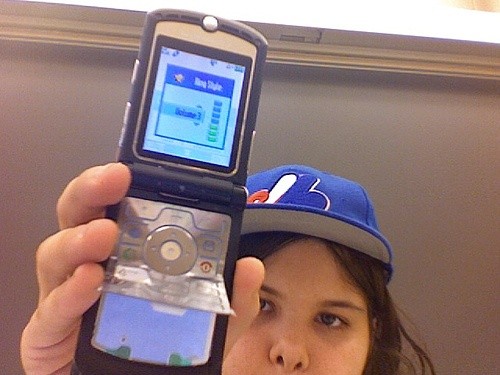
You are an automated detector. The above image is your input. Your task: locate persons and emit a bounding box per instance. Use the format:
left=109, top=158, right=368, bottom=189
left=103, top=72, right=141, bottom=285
left=20, top=161, right=435, bottom=375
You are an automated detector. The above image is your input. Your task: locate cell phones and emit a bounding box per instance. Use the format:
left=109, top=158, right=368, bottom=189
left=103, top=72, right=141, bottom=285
left=73, top=8, right=268, bottom=374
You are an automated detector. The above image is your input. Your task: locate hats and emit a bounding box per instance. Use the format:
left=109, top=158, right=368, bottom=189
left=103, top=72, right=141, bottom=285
left=240, top=165, right=392, bottom=265
